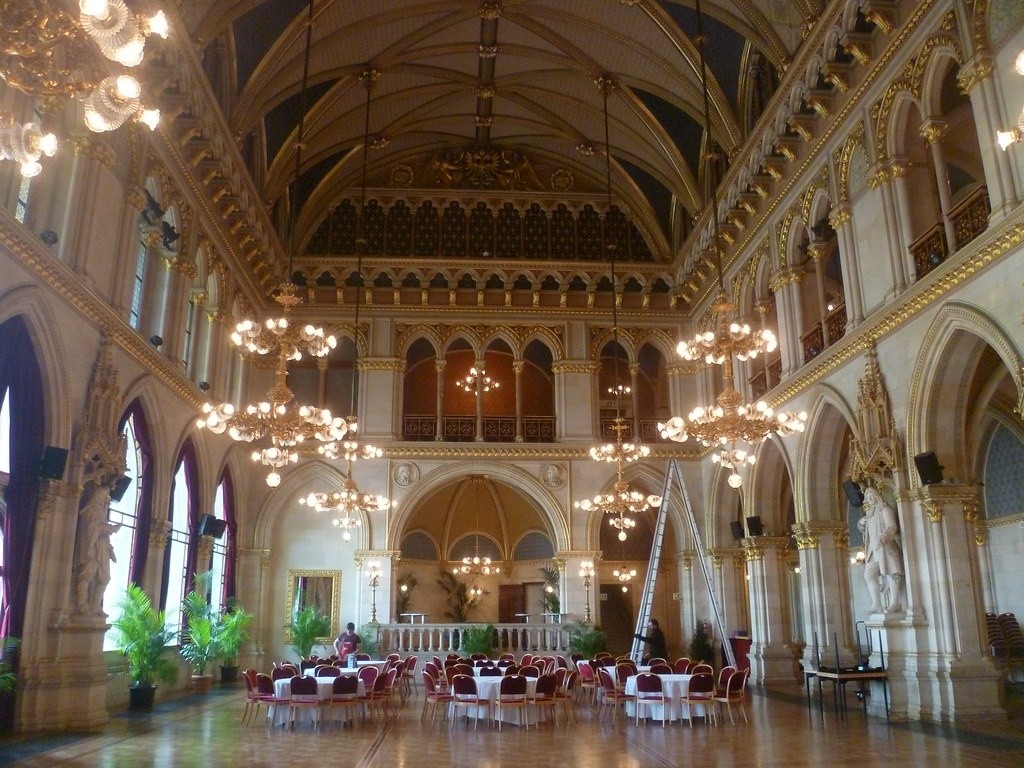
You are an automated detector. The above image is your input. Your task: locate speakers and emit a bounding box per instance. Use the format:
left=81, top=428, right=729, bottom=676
left=38, top=446, right=69, bottom=481
left=746, top=516, right=763, bottom=536
left=197, top=514, right=227, bottom=540
left=109, top=475, right=132, bottom=503
left=842, top=479, right=865, bottom=508
left=914, top=451, right=944, bottom=486
left=730, top=521, right=744, bottom=541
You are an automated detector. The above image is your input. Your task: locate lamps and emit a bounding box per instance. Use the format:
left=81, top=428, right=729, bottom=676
left=653, top=320, right=808, bottom=495
left=452, top=557, right=507, bottom=596
left=301, top=413, right=396, bottom=542
left=198, top=317, right=348, bottom=489
left=453, top=363, right=497, bottom=401
left=0, top=1, right=170, bottom=179
left=996, top=46, right=1024, bottom=157
left=573, top=440, right=663, bottom=544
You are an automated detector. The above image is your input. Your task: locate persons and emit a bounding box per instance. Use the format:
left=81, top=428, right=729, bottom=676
left=633, top=619, right=669, bottom=666
left=333, top=622, right=362, bottom=662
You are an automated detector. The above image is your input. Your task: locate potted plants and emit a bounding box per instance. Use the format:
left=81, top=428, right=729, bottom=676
left=112, top=583, right=726, bottom=715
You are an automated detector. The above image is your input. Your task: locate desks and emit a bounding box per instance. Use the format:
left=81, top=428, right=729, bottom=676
left=472, top=659, right=503, bottom=666
left=344, top=661, right=387, bottom=676
left=797, top=630, right=893, bottom=728
left=576, top=659, right=593, bottom=669
left=469, top=667, right=511, bottom=676
left=625, top=676, right=726, bottom=718
left=301, top=667, right=363, bottom=678
left=597, top=665, right=650, bottom=705
left=449, top=676, right=557, bottom=724
left=271, top=677, right=365, bottom=726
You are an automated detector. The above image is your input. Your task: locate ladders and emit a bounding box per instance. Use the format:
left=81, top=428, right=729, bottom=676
left=629, top=457, right=737, bottom=668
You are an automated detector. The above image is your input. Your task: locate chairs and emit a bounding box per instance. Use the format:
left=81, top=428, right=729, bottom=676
left=244, top=653, right=750, bottom=732
